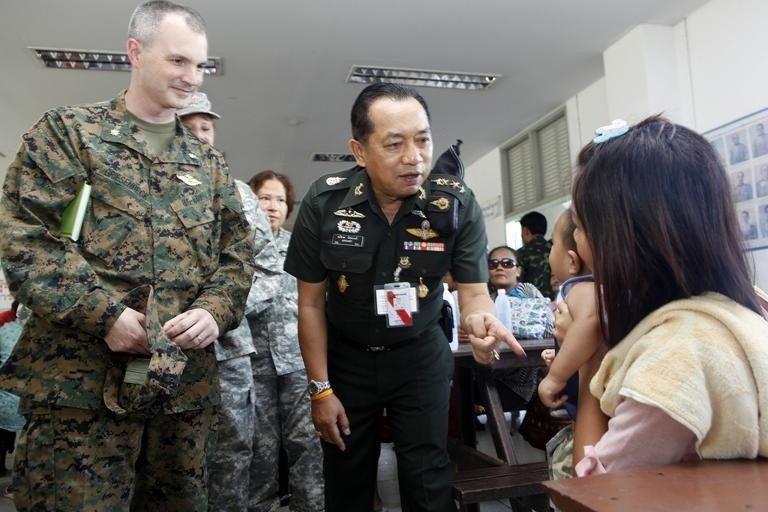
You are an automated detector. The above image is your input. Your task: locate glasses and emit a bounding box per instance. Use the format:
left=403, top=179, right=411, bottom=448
left=488, top=258, right=521, bottom=270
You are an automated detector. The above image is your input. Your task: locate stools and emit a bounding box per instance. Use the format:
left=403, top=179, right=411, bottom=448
left=445, top=459, right=561, bottom=511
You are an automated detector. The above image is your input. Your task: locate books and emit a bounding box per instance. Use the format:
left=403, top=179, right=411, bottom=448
left=60, top=183, right=92, bottom=242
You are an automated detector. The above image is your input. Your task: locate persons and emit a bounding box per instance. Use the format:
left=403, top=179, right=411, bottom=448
left=283, top=80, right=527, bottom=512
left=175, top=90, right=283, bottom=512
left=245, top=168, right=327, bottom=512
left=1, top=298, right=33, bottom=500
left=440, top=109, right=768, bottom=511
left=0, top=0, right=255, bottom=511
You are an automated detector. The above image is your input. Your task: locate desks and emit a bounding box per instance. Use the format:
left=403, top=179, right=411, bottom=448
left=447, top=335, right=561, bottom=465
left=542, top=460, right=768, bottom=512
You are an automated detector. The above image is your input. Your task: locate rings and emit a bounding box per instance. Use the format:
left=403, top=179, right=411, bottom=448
left=197, top=334, right=204, bottom=343
left=314, top=430, right=322, bottom=437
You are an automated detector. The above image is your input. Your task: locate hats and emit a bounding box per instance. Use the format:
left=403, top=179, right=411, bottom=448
left=176, top=91, right=221, bottom=121
left=103, top=284, right=186, bottom=418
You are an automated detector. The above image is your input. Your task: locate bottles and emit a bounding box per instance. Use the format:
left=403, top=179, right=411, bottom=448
left=493, top=288, right=513, bottom=335
left=441, top=282, right=458, bottom=353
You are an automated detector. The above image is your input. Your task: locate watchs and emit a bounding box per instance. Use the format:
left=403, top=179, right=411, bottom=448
left=307, top=378, right=332, bottom=396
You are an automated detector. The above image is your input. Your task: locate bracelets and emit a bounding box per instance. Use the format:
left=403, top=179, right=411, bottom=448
left=309, top=388, right=333, bottom=401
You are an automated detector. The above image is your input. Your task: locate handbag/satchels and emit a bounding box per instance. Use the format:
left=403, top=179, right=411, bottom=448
left=504, top=296, right=551, bottom=339
